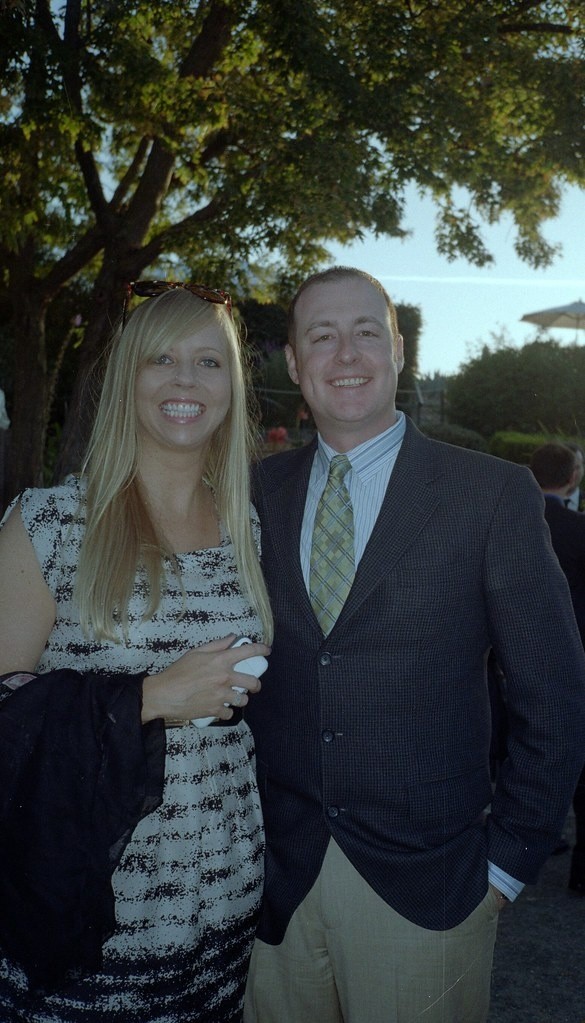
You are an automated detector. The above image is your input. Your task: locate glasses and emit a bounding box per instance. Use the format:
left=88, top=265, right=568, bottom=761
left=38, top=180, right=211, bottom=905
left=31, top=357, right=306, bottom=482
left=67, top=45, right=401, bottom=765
left=121, top=279, right=233, bottom=332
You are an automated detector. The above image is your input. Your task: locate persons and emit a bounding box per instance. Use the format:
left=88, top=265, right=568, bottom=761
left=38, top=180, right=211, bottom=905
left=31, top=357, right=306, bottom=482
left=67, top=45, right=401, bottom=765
left=244, top=266, right=585, bottom=1023
left=521, top=444, right=585, bottom=650
left=0, top=289, right=268, bottom=1023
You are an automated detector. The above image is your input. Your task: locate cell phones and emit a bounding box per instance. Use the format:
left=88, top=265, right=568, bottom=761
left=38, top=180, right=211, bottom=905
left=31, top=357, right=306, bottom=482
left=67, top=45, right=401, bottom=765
left=190, top=635, right=269, bottom=729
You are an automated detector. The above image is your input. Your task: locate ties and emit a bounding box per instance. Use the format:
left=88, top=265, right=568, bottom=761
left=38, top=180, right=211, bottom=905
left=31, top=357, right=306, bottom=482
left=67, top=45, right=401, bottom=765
left=563, top=500, right=571, bottom=508
left=308, top=454, right=353, bottom=640
